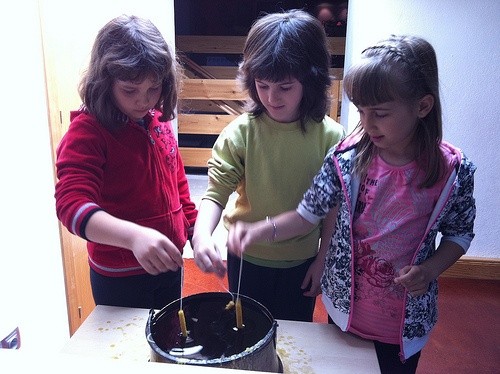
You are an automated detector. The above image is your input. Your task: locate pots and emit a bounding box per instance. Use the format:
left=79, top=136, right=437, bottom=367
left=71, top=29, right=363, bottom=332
left=145, top=291, right=280, bottom=373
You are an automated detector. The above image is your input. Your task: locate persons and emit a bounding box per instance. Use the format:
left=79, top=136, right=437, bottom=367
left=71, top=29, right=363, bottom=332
left=228, top=34, right=477, bottom=374
left=191, top=5, right=348, bottom=322
left=53, top=13, right=206, bottom=308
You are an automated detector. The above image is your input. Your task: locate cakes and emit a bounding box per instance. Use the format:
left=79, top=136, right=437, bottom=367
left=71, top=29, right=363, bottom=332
left=146, top=292, right=279, bottom=374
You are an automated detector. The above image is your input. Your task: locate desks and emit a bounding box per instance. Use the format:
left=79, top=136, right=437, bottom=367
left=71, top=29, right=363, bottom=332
left=67, top=304, right=384, bottom=374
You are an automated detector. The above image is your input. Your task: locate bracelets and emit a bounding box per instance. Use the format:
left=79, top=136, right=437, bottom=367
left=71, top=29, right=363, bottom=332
left=267, top=216, right=278, bottom=241
